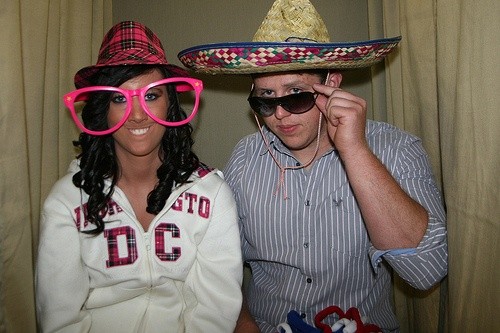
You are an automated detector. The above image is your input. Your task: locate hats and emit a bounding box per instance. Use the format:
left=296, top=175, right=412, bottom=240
left=177, top=0, right=403, bottom=74
left=73, top=20, right=192, bottom=96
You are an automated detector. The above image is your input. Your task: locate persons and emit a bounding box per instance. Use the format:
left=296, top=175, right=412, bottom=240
left=34, top=20, right=243, bottom=333
left=178, top=0, right=448, bottom=333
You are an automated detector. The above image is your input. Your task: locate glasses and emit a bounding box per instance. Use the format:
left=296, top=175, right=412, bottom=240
left=247, top=74, right=326, bottom=116
left=64, top=77, right=203, bottom=133
